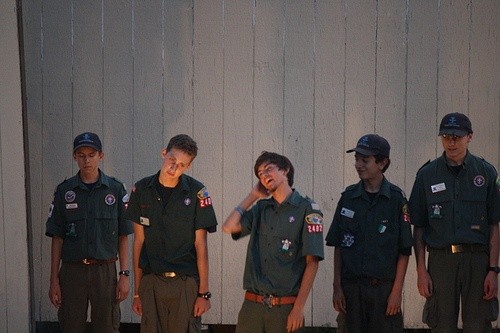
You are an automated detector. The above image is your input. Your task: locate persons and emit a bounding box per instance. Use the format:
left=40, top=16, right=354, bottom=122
left=222, top=151, right=324, bottom=333
left=125, top=134, right=217, bottom=333
left=325, top=134, right=413, bottom=333
left=410, top=112, right=500, bottom=333
left=44, top=132, right=134, bottom=333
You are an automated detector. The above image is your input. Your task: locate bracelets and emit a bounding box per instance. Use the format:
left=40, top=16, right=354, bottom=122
left=236, top=207, right=244, bottom=217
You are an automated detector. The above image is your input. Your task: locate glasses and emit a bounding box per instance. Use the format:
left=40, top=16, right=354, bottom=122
left=440, top=134, right=465, bottom=141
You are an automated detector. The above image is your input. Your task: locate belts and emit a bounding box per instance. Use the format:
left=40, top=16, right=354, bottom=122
left=80, top=257, right=118, bottom=265
left=245, top=292, right=296, bottom=305
left=142, top=269, right=198, bottom=277
left=441, top=243, right=483, bottom=253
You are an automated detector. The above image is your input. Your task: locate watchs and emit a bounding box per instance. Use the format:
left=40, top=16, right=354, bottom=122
left=119, top=270, right=129, bottom=276
left=489, top=266, right=499, bottom=273
left=197, top=292, right=211, bottom=299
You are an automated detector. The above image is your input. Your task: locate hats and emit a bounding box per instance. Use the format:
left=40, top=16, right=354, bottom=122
left=438, top=112, right=472, bottom=136
left=72, top=132, right=103, bottom=151
left=346, top=134, right=390, bottom=156
